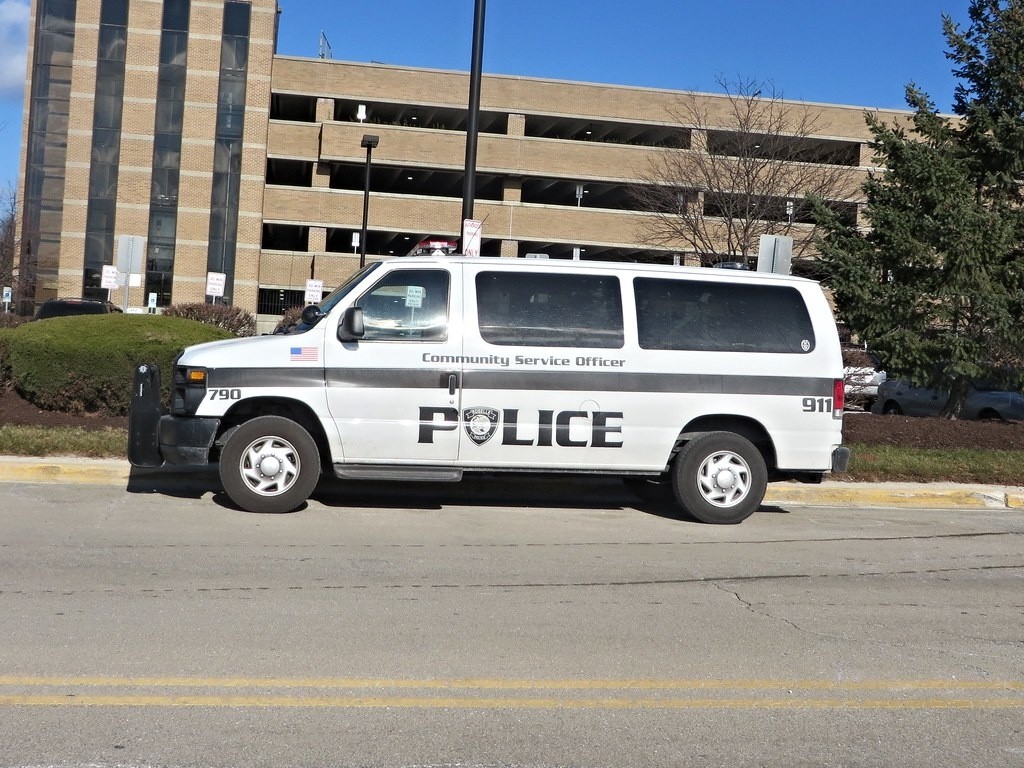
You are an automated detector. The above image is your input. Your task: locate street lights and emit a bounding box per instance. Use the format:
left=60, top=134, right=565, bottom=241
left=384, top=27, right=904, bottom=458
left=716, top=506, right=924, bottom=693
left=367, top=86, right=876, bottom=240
left=359, top=134, right=380, bottom=268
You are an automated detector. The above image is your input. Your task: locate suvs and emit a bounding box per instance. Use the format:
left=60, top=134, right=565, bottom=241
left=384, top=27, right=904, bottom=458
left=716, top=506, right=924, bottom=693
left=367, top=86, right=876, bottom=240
left=31, top=296, right=124, bottom=320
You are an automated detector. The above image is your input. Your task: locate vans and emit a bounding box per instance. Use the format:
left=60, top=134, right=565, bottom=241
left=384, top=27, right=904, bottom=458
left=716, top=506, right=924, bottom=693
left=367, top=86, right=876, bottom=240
left=126, top=255, right=852, bottom=525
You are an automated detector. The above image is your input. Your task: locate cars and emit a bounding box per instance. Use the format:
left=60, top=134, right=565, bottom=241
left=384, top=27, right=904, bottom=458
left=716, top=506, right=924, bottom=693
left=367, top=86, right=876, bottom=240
left=841, top=346, right=1024, bottom=422
